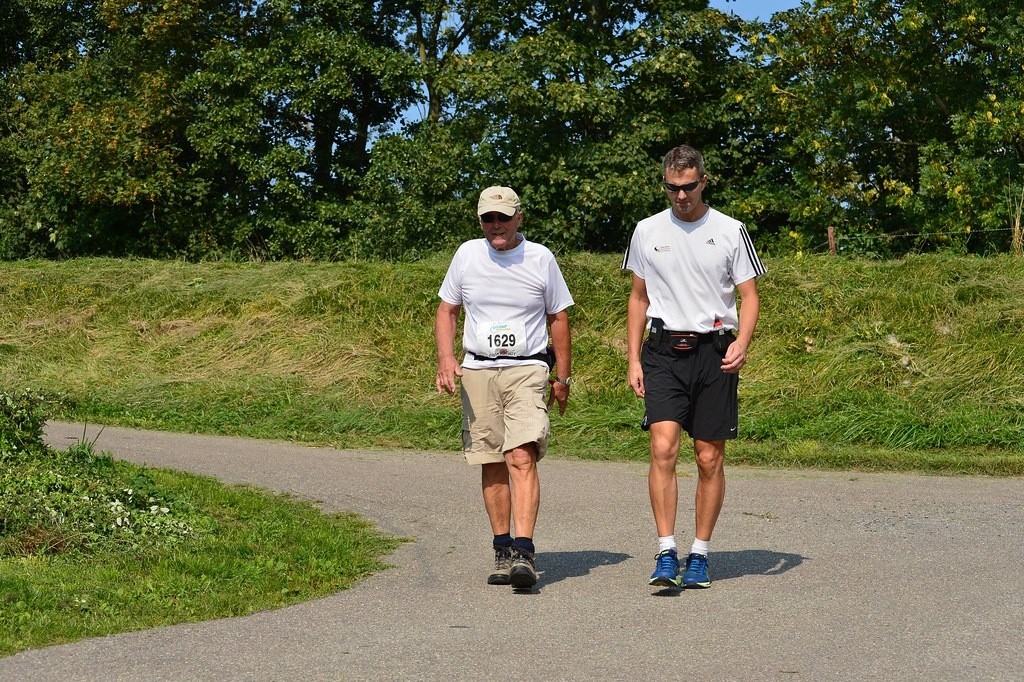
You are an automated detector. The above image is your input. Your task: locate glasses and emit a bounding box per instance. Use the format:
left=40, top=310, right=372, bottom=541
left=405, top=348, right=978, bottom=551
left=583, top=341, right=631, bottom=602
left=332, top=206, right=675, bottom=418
left=662, top=176, right=702, bottom=191
left=479, top=213, right=513, bottom=223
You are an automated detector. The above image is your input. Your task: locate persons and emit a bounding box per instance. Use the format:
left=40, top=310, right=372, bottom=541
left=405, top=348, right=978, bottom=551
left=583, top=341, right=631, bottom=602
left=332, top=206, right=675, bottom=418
left=620, top=146, right=767, bottom=589
left=434, top=187, right=575, bottom=589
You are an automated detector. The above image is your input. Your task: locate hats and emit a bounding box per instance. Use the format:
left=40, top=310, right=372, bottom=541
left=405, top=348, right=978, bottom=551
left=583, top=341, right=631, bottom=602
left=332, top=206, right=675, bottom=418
left=476, top=186, right=521, bottom=217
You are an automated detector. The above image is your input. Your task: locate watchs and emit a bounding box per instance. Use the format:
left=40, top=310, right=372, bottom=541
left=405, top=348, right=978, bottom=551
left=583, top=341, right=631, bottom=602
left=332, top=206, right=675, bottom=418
left=555, top=376, right=572, bottom=385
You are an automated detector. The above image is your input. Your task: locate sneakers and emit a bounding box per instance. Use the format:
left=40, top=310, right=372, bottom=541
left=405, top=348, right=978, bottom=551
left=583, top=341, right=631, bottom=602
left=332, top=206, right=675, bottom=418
left=488, top=542, right=514, bottom=585
left=647, top=547, right=682, bottom=587
left=509, top=544, right=537, bottom=588
left=681, top=552, right=709, bottom=588
